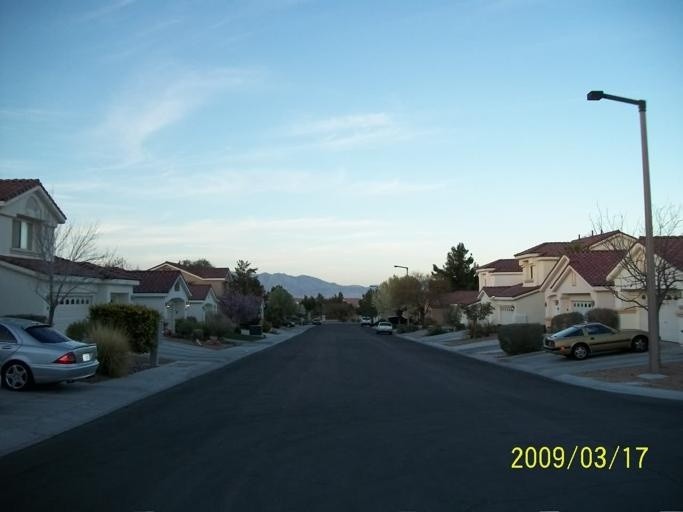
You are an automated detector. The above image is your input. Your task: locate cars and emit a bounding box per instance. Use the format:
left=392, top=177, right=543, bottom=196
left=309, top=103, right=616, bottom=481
left=376, top=322, right=393, bottom=335
left=0, top=317, right=101, bottom=391
left=541, top=322, right=649, bottom=360
left=287, top=315, right=303, bottom=324
left=311, top=317, right=321, bottom=325
left=280, top=318, right=294, bottom=328
left=360, top=317, right=373, bottom=327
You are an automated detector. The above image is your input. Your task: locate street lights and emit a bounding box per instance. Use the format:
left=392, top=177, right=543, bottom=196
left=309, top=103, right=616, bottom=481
left=394, top=265, right=410, bottom=330
left=370, top=285, right=379, bottom=294
left=584, top=89, right=664, bottom=373
